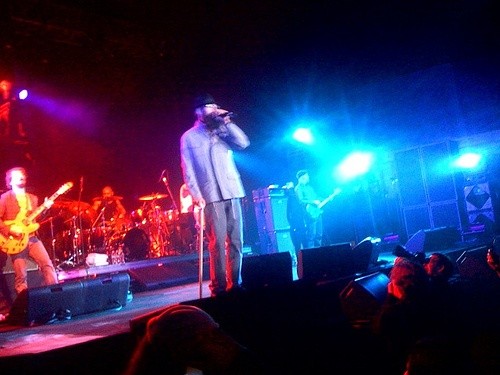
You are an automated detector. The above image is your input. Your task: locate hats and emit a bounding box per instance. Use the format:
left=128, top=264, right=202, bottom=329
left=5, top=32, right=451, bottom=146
left=192, top=95, right=215, bottom=111
left=296, top=169, right=308, bottom=179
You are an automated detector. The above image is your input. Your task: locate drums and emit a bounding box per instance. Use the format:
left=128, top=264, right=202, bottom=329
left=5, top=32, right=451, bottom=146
left=108, top=227, right=151, bottom=262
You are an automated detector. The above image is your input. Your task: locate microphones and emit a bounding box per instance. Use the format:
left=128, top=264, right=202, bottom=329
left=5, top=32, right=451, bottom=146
left=158, top=170, right=164, bottom=183
left=210, top=112, right=234, bottom=120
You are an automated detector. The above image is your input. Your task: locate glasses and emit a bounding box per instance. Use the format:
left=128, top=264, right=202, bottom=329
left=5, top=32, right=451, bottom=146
left=204, top=103, right=221, bottom=109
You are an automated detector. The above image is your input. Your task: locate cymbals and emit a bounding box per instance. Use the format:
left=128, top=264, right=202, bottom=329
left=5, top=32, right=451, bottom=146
left=139, top=195, right=168, bottom=200
left=93, top=196, right=123, bottom=201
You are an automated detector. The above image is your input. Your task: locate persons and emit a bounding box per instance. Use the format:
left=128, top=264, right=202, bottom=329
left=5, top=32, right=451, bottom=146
left=93, top=186, right=126, bottom=220
left=0, top=167, right=60, bottom=294
left=128, top=236, right=500, bottom=375
left=181, top=93, right=251, bottom=297
left=294, top=169, right=324, bottom=248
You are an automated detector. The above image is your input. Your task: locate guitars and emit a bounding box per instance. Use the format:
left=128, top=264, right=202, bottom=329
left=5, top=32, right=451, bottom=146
left=0, top=180, right=74, bottom=255
left=306, top=187, right=343, bottom=220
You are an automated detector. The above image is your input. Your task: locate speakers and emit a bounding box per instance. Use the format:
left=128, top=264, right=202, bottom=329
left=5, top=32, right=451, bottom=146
left=351, top=236, right=383, bottom=269
left=404, top=225, right=457, bottom=253
left=26, top=272, right=130, bottom=322
left=238, top=252, right=293, bottom=287
left=295, top=243, right=358, bottom=282
left=254, top=198, right=299, bottom=281
